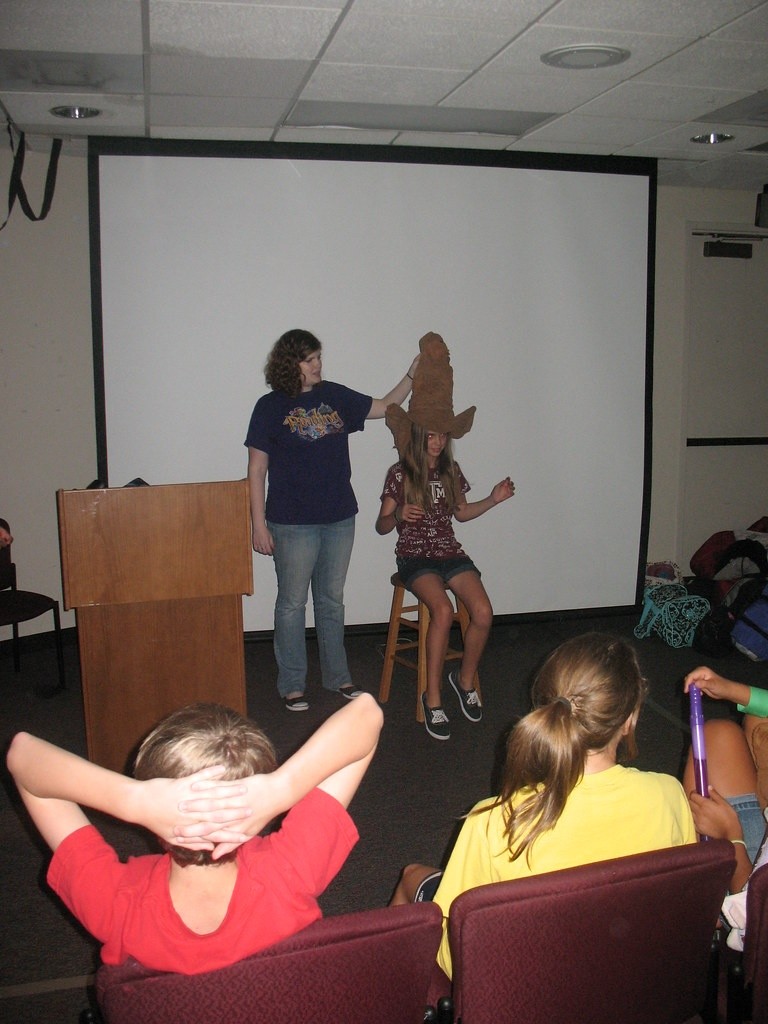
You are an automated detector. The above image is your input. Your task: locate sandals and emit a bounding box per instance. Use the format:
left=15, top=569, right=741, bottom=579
left=284, top=694, right=309, bottom=711
left=332, top=686, right=364, bottom=700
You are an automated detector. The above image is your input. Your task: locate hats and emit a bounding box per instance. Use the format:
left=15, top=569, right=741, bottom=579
left=385, top=331, right=477, bottom=461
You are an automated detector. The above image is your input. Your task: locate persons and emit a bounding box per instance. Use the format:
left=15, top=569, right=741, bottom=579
left=374, top=395, right=516, bottom=741
left=389, top=631, right=696, bottom=1007
left=684, top=664, right=768, bottom=952
left=8, top=689, right=385, bottom=975
left=244, top=328, right=422, bottom=711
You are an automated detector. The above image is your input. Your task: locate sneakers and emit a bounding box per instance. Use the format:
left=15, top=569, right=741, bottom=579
left=422, top=691, right=451, bottom=740
left=448, top=670, right=482, bottom=723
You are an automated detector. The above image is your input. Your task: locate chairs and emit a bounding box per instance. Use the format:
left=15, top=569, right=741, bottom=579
left=0, top=517, right=65, bottom=688
left=80, top=838, right=768, bottom=1024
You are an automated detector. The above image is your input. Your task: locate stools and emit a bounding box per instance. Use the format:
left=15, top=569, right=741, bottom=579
left=379, top=572, right=483, bottom=723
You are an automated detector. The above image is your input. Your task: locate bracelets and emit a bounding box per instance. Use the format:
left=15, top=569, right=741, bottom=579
left=729, top=838, right=748, bottom=850
left=392, top=506, right=400, bottom=523
left=406, top=373, right=414, bottom=381
